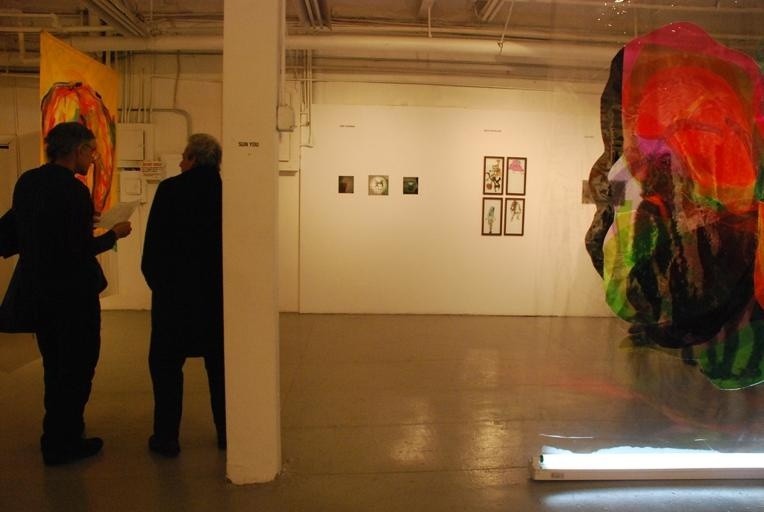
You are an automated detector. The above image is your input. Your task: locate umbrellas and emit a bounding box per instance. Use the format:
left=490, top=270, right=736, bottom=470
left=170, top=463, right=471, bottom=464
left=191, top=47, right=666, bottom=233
left=43, top=437, right=103, bottom=467
left=149, top=435, right=181, bottom=458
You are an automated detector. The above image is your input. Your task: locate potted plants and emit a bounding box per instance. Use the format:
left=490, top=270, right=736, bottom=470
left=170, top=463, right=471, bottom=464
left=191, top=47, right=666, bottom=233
left=506, top=157, right=526, bottom=196
left=483, top=156, right=503, bottom=195
left=482, top=197, right=502, bottom=235
left=504, top=198, right=525, bottom=236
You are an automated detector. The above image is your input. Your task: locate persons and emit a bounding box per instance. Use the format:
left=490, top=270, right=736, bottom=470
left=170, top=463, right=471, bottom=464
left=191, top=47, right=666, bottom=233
left=138, top=131, right=229, bottom=456
left=9, top=121, right=133, bottom=465
